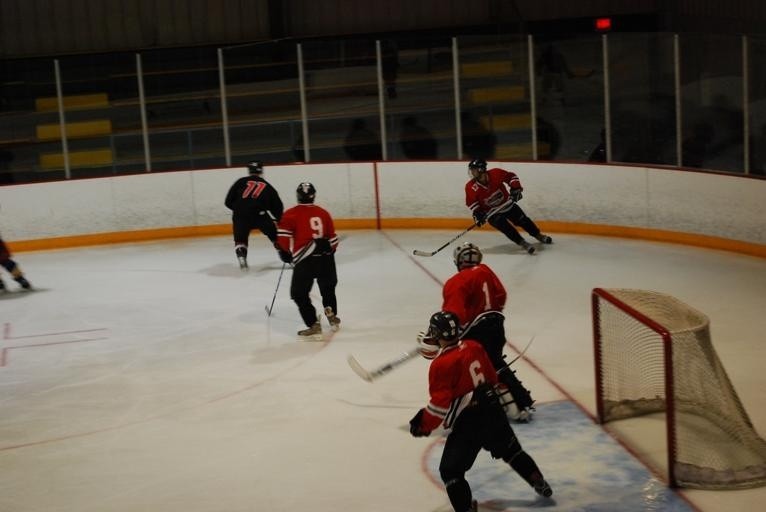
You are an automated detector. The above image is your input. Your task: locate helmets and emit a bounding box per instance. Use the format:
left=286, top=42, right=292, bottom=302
left=469, top=159, right=487, bottom=173
left=428, top=311, right=458, bottom=342
left=453, top=243, right=482, bottom=272
left=296, top=183, right=316, bottom=201
left=249, top=160, right=263, bottom=174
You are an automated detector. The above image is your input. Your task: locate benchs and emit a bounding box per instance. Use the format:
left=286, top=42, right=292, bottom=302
left=2, top=33, right=552, bottom=183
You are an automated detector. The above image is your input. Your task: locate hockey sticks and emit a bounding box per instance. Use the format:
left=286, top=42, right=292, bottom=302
left=414, top=198, right=513, bottom=258
left=494, top=339, right=535, bottom=367
left=343, top=348, right=420, bottom=384
left=264, top=261, right=287, bottom=317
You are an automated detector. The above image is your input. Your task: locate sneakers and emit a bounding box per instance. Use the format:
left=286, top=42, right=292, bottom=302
left=535, top=481, right=553, bottom=497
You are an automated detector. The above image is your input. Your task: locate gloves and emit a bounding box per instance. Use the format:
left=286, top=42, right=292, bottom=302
left=416, top=332, right=441, bottom=360
left=279, top=249, right=293, bottom=264
left=508, top=189, right=522, bottom=202
left=410, top=411, right=430, bottom=437
left=473, top=211, right=488, bottom=227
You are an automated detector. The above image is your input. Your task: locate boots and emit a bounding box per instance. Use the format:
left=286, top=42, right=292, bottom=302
left=298, top=315, right=322, bottom=336
left=15, top=275, right=30, bottom=289
left=324, top=307, right=341, bottom=325
left=536, top=234, right=551, bottom=244
left=518, top=240, right=535, bottom=254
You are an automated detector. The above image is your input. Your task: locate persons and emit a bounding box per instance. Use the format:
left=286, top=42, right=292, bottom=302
left=430, top=241, right=535, bottom=425
left=276, top=182, right=342, bottom=338
left=402, top=313, right=556, bottom=511
left=1, top=239, right=32, bottom=298
left=223, top=158, right=293, bottom=269
left=463, top=158, right=552, bottom=257
